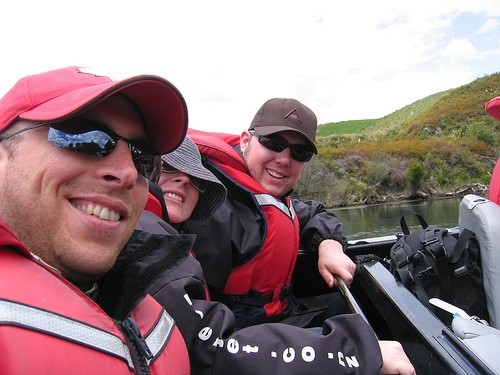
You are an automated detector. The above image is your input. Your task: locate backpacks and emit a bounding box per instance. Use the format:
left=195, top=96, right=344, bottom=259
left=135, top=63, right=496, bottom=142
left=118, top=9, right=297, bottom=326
left=389, top=211, right=490, bottom=328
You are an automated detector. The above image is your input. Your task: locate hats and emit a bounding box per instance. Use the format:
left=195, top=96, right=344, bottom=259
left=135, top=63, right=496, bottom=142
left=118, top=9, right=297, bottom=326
left=159, top=135, right=228, bottom=222
left=248, top=97, right=318, bottom=156
left=0, top=65, right=188, bottom=154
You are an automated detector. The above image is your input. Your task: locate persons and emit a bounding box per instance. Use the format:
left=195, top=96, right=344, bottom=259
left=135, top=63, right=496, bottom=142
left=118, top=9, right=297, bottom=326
left=145, top=134, right=418, bottom=375
left=186, top=98, right=356, bottom=328
left=0, top=64, right=214, bottom=375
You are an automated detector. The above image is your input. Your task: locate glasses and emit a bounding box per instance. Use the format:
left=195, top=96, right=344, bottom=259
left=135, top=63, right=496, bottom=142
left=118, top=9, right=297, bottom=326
left=160, top=161, right=208, bottom=194
left=0, top=119, right=162, bottom=185
left=249, top=129, right=314, bottom=163
